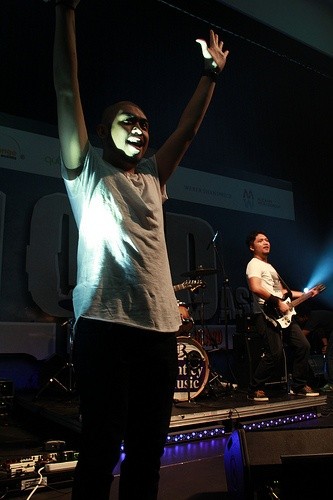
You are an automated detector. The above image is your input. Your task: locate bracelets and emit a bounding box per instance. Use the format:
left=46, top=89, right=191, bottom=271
left=203, top=68, right=218, bottom=82
left=323, top=345, right=326, bottom=346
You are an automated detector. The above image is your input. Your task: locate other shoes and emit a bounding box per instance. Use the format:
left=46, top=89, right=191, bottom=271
left=290, top=385, right=319, bottom=397
left=246, top=390, right=269, bottom=401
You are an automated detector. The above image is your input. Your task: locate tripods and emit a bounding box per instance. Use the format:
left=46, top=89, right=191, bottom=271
left=36, top=317, right=75, bottom=399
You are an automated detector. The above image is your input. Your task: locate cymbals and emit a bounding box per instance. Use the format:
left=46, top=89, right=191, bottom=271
left=59, top=298, right=74, bottom=312
left=185, top=301, right=209, bottom=305
left=182, top=267, right=218, bottom=277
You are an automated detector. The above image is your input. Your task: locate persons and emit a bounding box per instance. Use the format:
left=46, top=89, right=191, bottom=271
left=246, top=233, right=320, bottom=400
left=296, top=311, right=328, bottom=395
left=50, top=0, right=229, bottom=500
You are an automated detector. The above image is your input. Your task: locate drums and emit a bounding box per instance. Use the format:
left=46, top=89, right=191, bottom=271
left=171, top=336, right=209, bottom=402
left=186, top=327, right=212, bottom=347
left=175, top=301, right=195, bottom=335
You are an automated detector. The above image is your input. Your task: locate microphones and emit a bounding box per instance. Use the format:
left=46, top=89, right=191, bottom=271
left=213, top=231, right=219, bottom=241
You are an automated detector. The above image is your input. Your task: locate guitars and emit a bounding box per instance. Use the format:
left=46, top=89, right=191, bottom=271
left=262, top=282, right=327, bottom=329
left=172, top=279, right=208, bottom=292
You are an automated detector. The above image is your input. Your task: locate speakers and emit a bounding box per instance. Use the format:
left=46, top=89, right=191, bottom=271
left=232, top=333, right=289, bottom=387
